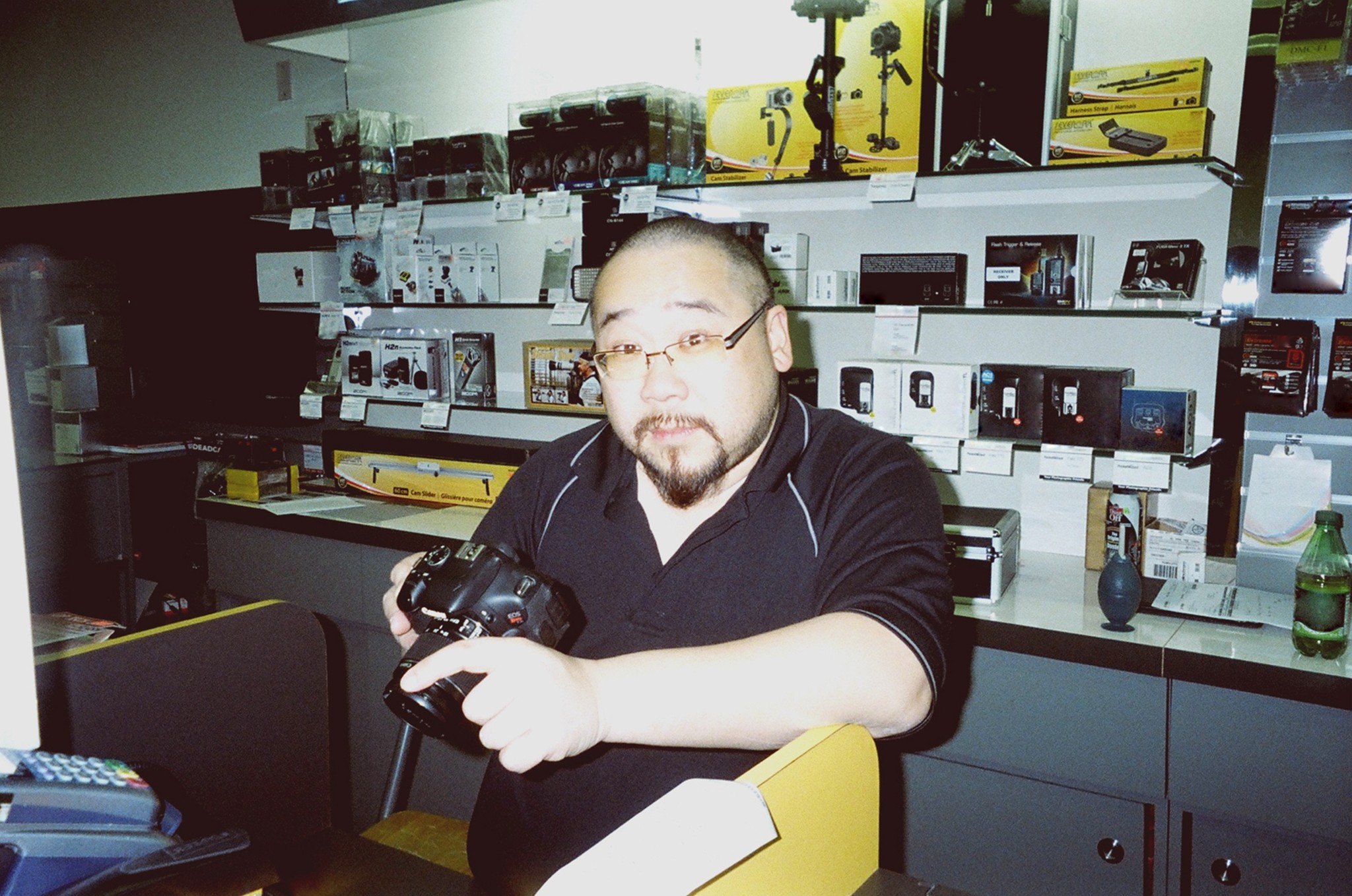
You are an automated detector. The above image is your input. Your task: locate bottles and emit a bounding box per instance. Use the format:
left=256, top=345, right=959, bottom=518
left=1291, top=510, right=1352, bottom=660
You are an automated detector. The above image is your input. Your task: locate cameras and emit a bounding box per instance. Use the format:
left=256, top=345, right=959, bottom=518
left=766, top=88, right=793, bottom=108
left=851, top=89, right=863, bottom=99
left=382, top=539, right=572, bottom=751
left=871, top=22, right=900, bottom=49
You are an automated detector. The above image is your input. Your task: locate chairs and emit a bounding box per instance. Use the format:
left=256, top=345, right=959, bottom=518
left=355, top=719, right=495, bottom=896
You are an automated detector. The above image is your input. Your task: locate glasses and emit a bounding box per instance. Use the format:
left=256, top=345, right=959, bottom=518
left=592, top=297, right=775, bottom=381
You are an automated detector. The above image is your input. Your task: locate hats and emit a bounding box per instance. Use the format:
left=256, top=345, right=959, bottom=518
left=580, top=351, right=594, bottom=361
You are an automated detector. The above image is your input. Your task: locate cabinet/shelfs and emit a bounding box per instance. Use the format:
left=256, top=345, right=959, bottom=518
left=251, top=155, right=1246, bottom=467
left=203, top=522, right=1352, bottom=896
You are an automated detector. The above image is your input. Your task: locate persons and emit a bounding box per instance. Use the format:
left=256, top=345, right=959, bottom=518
left=382, top=218, right=956, bottom=894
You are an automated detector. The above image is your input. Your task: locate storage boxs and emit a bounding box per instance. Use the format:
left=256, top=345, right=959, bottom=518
left=1049, top=106, right=1213, bottom=167
left=1066, top=57, right=1213, bottom=118
